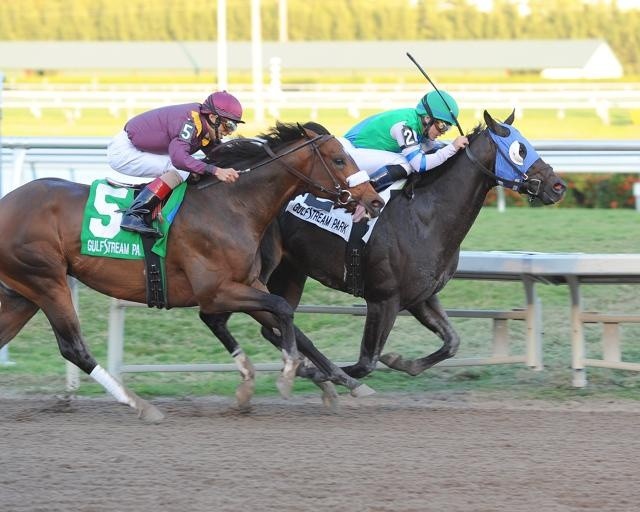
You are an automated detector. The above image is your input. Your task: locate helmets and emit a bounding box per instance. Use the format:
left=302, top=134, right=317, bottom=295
left=416, top=90, right=459, bottom=127
left=200, top=91, right=246, bottom=123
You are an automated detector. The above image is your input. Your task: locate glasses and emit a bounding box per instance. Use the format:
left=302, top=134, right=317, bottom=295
left=217, top=119, right=241, bottom=131
left=435, top=121, right=449, bottom=134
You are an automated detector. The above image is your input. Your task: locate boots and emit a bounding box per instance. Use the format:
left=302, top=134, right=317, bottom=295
left=120, top=178, right=172, bottom=237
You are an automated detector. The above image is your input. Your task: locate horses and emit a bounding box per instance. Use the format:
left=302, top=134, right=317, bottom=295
left=0, top=119, right=385, bottom=425
left=197, top=106, right=566, bottom=407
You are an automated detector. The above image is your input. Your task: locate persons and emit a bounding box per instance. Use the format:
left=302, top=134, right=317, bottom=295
left=336, top=90, right=469, bottom=194
left=106, top=92, right=244, bottom=238
